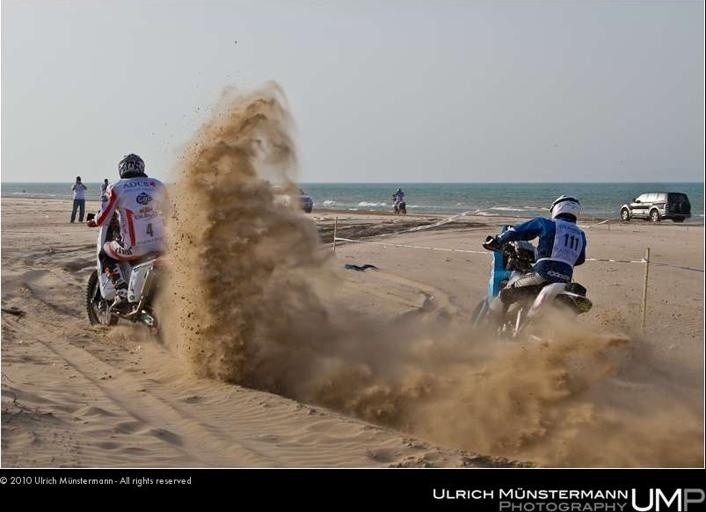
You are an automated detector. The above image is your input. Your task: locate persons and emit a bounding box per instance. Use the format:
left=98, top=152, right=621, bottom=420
left=70, top=176, right=88, bottom=223
left=99, top=178, right=110, bottom=208
left=391, top=187, right=406, bottom=213
left=86, top=152, right=175, bottom=312
left=481, top=194, right=589, bottom=332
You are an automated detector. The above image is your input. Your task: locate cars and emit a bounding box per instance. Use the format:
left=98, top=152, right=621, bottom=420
left=272, top=186, right=313, bottom=213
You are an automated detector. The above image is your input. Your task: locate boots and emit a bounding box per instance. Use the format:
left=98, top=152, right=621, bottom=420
left=105, top=264, right=128, bottom=313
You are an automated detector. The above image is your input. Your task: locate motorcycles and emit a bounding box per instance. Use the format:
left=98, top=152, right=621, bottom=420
left=466, top=224, right=592, bottom=347
left=391, top=198, right=406, bottom=215
left=86, top=213, right=173, bottom=347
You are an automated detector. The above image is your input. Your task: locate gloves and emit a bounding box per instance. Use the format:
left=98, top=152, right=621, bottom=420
left=87, top=219, right=98, bottom=227
left=483, top=236, right=500, bottom=252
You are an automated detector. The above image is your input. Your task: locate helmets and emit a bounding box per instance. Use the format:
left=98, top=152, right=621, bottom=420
left=119, top=154, right=145, bottom=178
left=550, top=194, right=583, bottom=220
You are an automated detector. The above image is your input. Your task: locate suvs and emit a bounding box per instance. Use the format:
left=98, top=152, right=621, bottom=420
left=620, top=192, right=692, bottom=222
